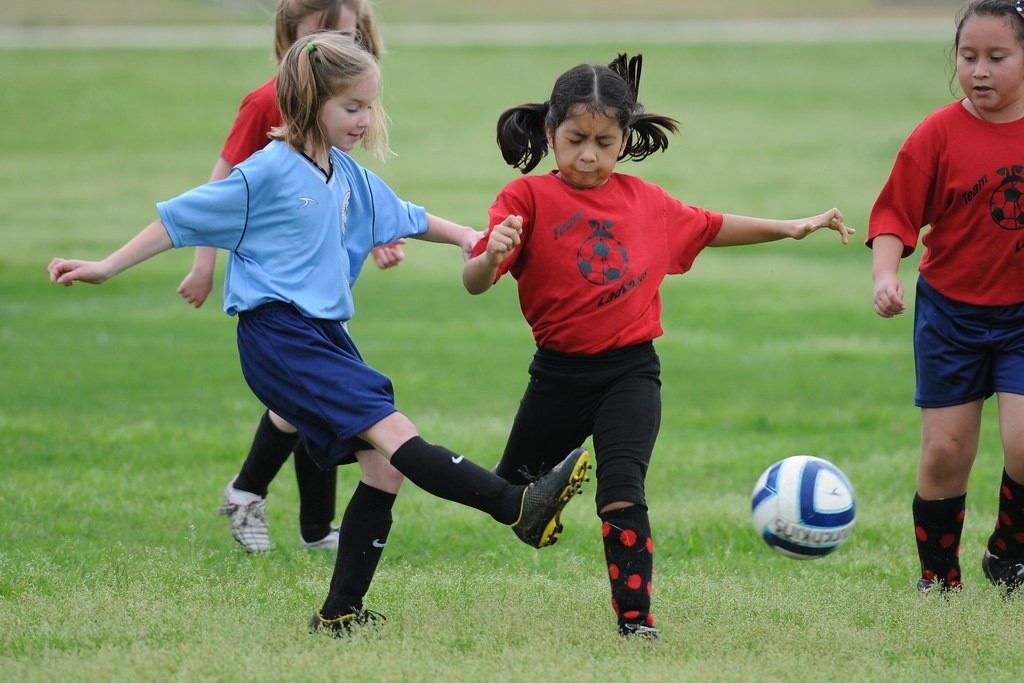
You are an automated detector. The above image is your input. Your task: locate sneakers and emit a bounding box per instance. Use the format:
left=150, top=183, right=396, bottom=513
left=510, top=449, right=591, bottom=549
left=618, top=624, right=659, bottom=642
left=917, top=581, right=962, bottom=610
left=300, top=526, right=339, bottom=550
left=983, top=547, right=1024, bottom=601
left=307, top=609, right=386, bottom=640
left=216, top=476, right=271, bottom=552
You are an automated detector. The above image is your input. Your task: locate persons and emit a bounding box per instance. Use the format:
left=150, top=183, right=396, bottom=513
left=49, top=32, right=593, bottom=638
left=864, top=0, right=1024, bottom=599
left=178, top=0, right=403, bottom=554
left=463, top=54, right=855, bottom=640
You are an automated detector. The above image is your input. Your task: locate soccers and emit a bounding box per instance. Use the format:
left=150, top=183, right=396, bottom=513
left=750, top=454, right=855, bottom=562
